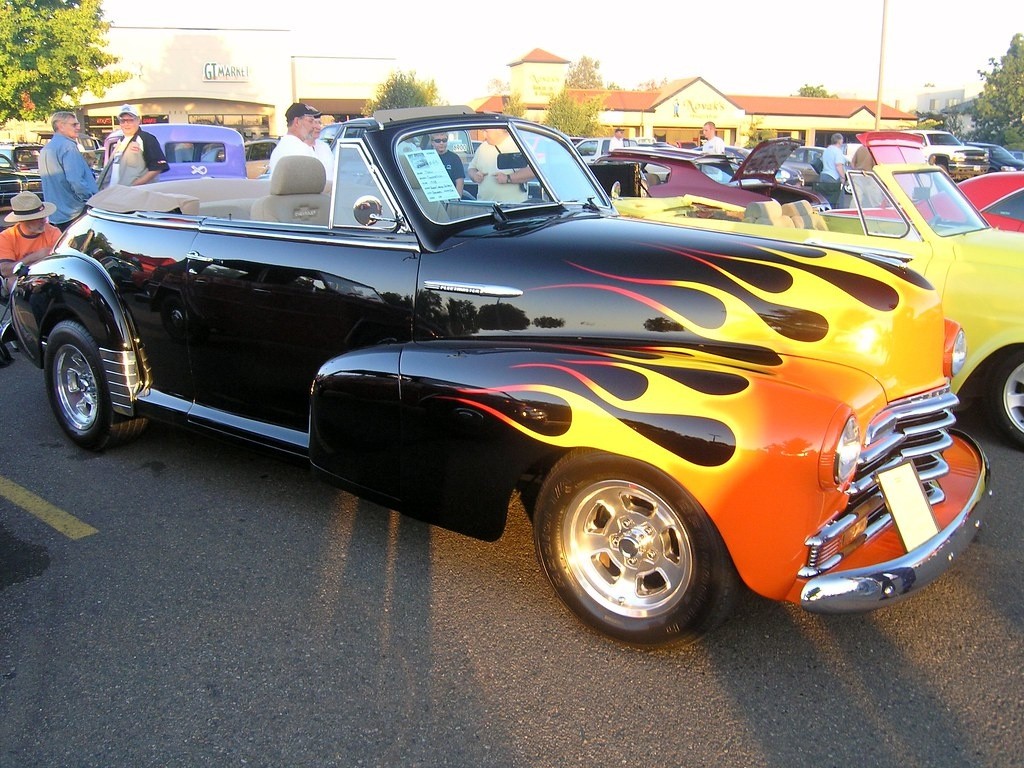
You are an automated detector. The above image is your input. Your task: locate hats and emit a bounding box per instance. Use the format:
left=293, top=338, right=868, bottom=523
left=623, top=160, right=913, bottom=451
left=4, top=191, right=57, bottom=222
left=615, top=128, right=624, bottom=133
left=118, top=104, right=142, bottom=118
left=285, top=102, right=322, bottom=122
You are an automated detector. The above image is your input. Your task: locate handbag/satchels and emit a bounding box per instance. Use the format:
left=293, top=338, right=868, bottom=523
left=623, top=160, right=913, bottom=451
left=809, top=152, right=823, bottom=174
left=520, top=182, right=528, bottom=193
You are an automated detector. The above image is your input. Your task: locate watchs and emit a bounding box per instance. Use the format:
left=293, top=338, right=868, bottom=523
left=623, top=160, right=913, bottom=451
left=843, top=178, right=846, bottom=179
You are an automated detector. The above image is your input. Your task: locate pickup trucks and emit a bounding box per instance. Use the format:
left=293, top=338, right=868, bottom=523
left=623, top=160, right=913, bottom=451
left=575, top=138, right=654, bottom=164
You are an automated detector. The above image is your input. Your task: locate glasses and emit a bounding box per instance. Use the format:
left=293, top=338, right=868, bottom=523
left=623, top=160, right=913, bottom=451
left=64, top=122, right=79, bottom=128
left=433, top=139, right=447, bottom=143
left=119, top=119, right=135, bottom=124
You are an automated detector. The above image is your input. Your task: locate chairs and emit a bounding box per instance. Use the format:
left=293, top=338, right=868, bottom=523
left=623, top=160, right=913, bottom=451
left=402, top=156, right=450, bottom=227
left=744, top=200, right=796, bottom=229
left=784, top=200, right=828, bottom=231
left=253, top=154, right=332, bottom=227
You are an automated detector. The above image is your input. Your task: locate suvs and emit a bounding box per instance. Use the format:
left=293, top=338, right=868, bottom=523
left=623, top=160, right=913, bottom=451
left=897, top=130, right=990, bottom=181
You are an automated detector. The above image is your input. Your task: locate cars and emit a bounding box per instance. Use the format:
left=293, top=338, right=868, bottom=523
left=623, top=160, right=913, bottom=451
left=965, top=143, right=1024, bottom=172
left=586, top=136, right=832, bottom=213
left=781, top=146, right=827, bottom=186
left=0, top=143, right=45, bottom=212
left=103, top=123, right=247, bottom=188
left=692, top=146, right=751, bottom=165
left=1008, top=151, right=1024, bottom=161
left=200, top=139, right=281, bottom=179
left=827, top=130, right=1024, bottom=232
left=608, top=160, right=1024, bottom=450
left=8, top=114, right=993, bottom=646
left=29, top=129, right=104, bottom=151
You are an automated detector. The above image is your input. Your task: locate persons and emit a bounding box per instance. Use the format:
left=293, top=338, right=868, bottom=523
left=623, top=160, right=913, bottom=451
left=609, top=128, right=625, bottom=152
left=269, top=103, right=337, bottom=180
left=468, top=129, right=536, bottom=203
left=809, top=133, right=877, bottom=210
left=37, top=112, right=98, bottom=231
left=108, top=104, right=170, bottom=187
left=702, top=122, right=725, bottom=182
left=0, top=191, right=62, bottom=300
left=429, top=131, right=465, bottom=201
